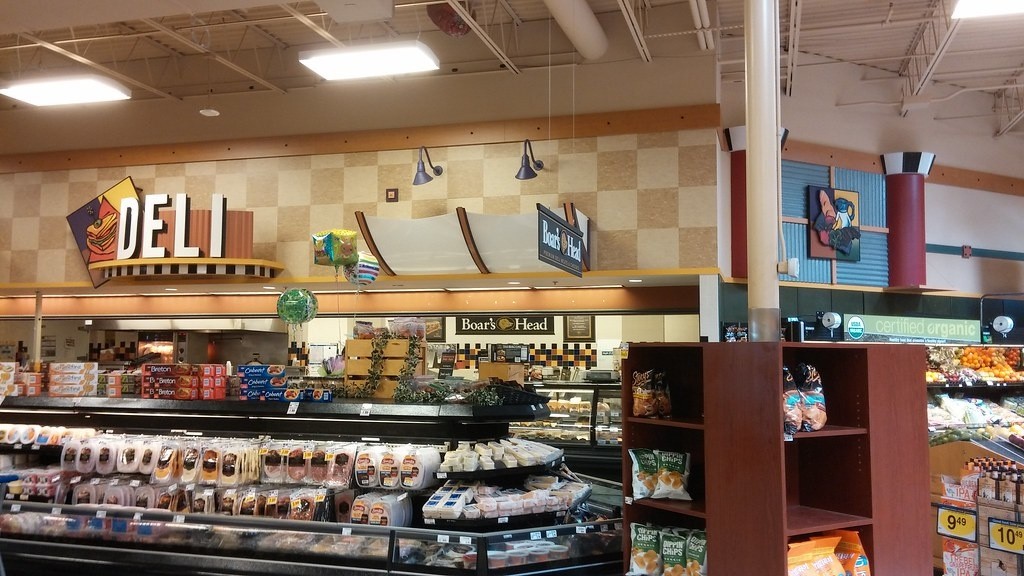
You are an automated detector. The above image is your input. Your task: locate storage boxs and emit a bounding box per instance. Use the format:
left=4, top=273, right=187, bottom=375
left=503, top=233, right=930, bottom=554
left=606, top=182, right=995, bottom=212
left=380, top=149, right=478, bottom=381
left=0, top=361, right=332, bottom=395
left=488, top=344, right=530, bottom=363
left=939, top=467, right=1024, bottom=576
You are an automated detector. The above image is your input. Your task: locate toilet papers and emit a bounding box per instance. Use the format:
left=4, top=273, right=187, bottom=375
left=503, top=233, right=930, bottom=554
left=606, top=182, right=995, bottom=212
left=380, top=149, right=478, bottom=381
left=993, top=316, right=1014, bottom=334
left=823, top=311, right=841, bottom=330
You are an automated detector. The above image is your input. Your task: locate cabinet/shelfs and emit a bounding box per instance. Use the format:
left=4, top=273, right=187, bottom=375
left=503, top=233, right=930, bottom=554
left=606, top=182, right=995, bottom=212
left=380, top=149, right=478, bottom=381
left=345, top=338, right=427, bottom=400
left=0, top=395, right=621, bottom=576
left=620, top=341, right=933, bottom=576
left=507, top=380, right=623, bottom=484
left=926, top=380, right=1024, bottom=437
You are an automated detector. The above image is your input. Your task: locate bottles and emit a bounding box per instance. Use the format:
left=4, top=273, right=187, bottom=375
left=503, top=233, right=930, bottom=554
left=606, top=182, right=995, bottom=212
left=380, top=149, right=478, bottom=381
left=966, top=455, right=1024, bottom=505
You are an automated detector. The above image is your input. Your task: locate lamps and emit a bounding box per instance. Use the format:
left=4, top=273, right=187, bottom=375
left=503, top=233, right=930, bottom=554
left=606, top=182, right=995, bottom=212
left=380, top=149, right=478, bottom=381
left=412, top=146, right=443, bottom=186
left=515, top=139, right=543, bottom=180
left=298, top=7, right=440, bottom=81
left=0, top=22, right=132, bottom=108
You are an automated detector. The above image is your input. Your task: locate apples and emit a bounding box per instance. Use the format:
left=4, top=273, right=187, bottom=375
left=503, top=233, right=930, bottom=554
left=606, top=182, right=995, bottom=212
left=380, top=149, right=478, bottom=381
left=1002, top=348, right=1021, bottom=366
left=1003, top=364, right=1024, bottom=382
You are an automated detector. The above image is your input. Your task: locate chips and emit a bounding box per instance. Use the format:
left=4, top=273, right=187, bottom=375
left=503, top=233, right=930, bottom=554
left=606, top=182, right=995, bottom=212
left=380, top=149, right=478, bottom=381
left=638, top=468, right=685, bottom=490
left=634, top=549, right=701, bottom=576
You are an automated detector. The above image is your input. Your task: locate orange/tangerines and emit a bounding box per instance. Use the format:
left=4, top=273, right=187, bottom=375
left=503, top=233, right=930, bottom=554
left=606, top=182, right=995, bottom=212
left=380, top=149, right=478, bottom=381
left=961, top=348, right=995, bottom=368
left=975, top=365, right=1013, bottom=382
left=928, top=428, right=990, bottom=446
